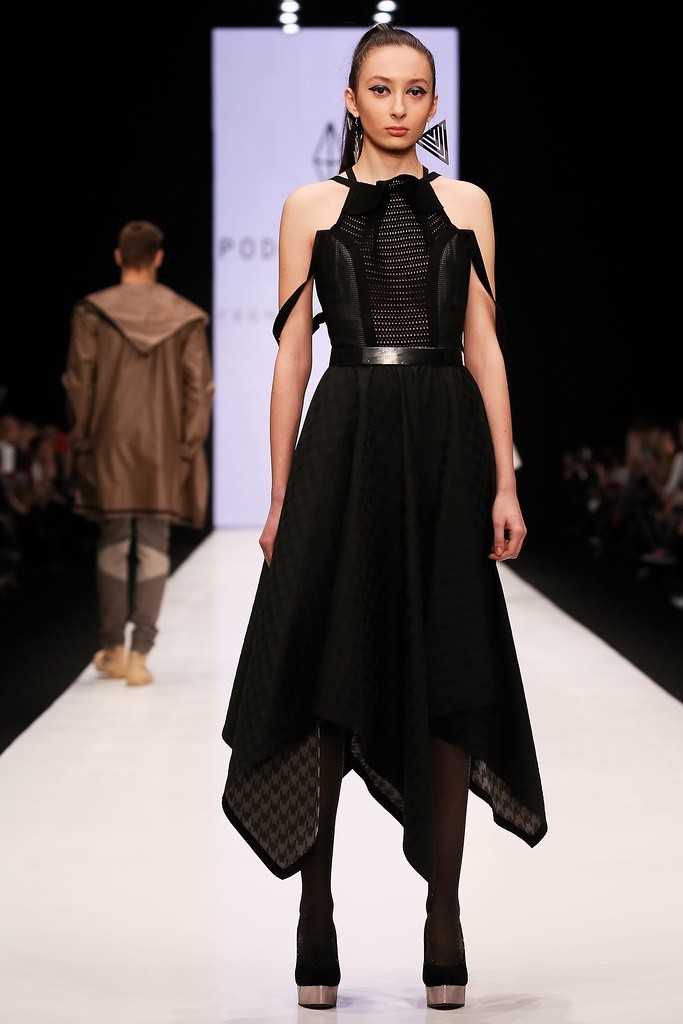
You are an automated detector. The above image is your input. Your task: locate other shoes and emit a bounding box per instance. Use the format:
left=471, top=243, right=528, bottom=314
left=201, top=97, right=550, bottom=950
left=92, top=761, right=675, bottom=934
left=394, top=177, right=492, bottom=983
left=94, top=650, right=125, bottom=677
left=125, top=665, right=151, bottom=685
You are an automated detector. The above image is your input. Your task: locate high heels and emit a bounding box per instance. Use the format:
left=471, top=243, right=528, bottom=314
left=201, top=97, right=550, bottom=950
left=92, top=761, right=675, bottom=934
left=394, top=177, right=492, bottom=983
left=295, top=921, right=340, bottom=1010
left=423, top=919, right=468, bottom=1009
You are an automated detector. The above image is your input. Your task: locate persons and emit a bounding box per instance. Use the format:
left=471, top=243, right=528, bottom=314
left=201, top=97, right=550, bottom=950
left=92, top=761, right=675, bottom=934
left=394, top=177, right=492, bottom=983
left=561, top=426, right=683, bottom=611
left=62, top=220, right=215, bottom=684
left=220, top=23, right=549, bottom=1011
left=0, top=416, right=87, bottom=515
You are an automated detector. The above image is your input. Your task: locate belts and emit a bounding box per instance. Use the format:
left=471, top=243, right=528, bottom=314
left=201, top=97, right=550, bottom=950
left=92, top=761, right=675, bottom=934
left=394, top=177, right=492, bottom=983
left=328, top=345, right=464, bottom=365
